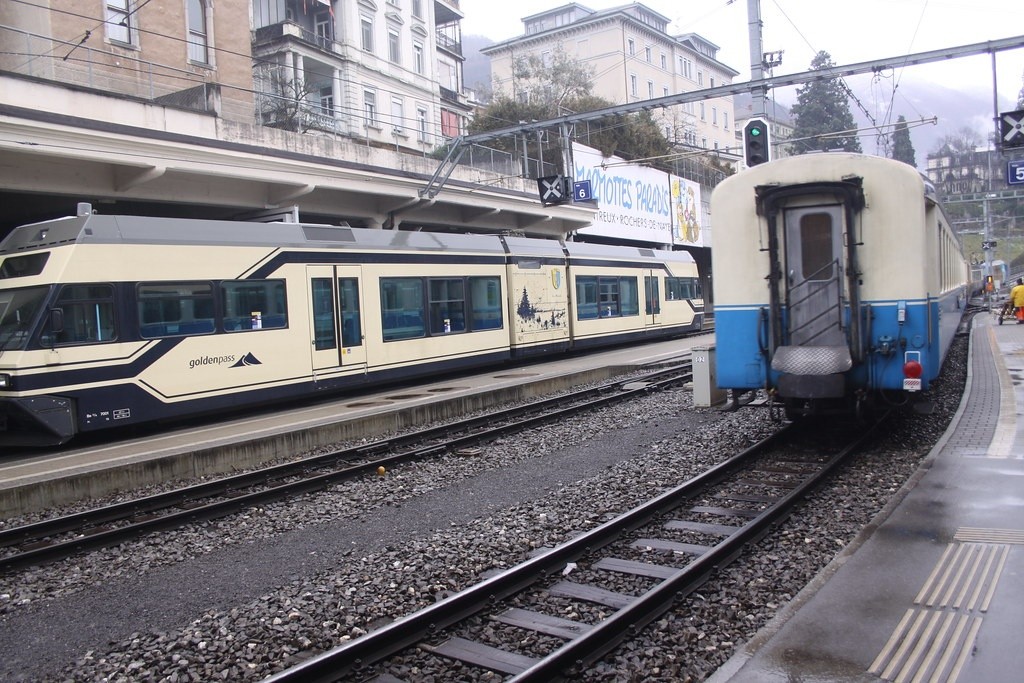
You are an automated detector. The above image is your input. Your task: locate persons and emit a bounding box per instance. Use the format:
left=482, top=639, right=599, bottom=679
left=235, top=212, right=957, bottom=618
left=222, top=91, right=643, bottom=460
left=1007, top=277, right=1024, bottom=324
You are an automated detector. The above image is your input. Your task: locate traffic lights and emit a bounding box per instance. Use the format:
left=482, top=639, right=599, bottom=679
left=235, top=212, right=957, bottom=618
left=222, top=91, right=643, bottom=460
left=742, top=117, right=772, bottom=170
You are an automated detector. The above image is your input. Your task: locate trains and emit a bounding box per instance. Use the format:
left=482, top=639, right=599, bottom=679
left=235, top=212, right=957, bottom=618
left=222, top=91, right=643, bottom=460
left=708, top=148, right=1008, bottom=430
left=0, top=213, right=710, bottom=453
